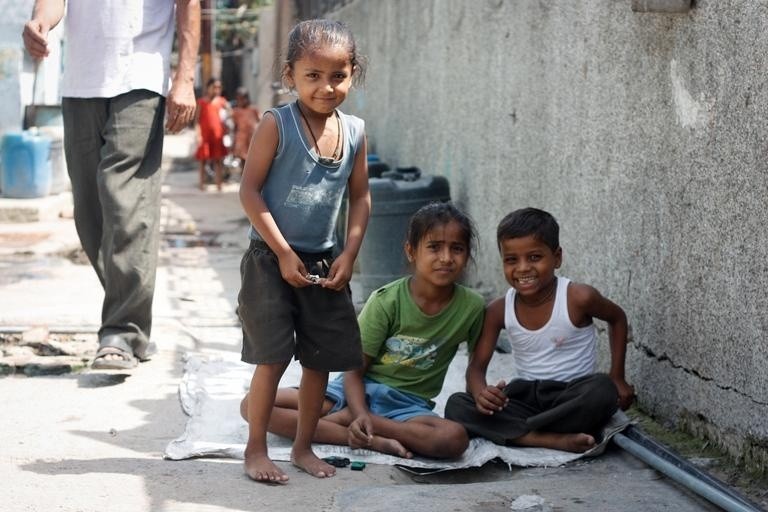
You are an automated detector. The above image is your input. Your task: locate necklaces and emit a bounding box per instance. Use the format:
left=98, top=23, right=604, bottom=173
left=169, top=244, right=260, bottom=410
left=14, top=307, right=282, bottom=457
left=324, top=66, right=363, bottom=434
left=296, top=99, right=340, bottom=163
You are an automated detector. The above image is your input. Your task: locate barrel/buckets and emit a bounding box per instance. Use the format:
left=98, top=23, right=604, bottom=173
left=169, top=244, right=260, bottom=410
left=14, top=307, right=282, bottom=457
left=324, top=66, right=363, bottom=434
left=0, top=126, right=53, bottom=199
left=334, top=153, right=389, bottom=272
left=357, top=166, right=450, bottom=303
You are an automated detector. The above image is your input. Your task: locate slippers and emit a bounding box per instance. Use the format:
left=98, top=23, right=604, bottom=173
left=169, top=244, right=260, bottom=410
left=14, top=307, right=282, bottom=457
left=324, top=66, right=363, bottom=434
left=92, top=335, right=142, bottom=377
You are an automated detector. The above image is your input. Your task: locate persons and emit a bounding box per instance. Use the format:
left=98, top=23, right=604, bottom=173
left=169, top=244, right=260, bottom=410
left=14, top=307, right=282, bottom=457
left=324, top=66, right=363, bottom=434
left=230, top=87, right=260, bottom=178
left=236, top=19, right=371, bottom=482
left=193, top=77, right=232, bottom=190
left=22, top=0, right=202, bottom=377
left=241, top=202, right=487, bottom=460
left=444, top=207, right=634, bottom=453
